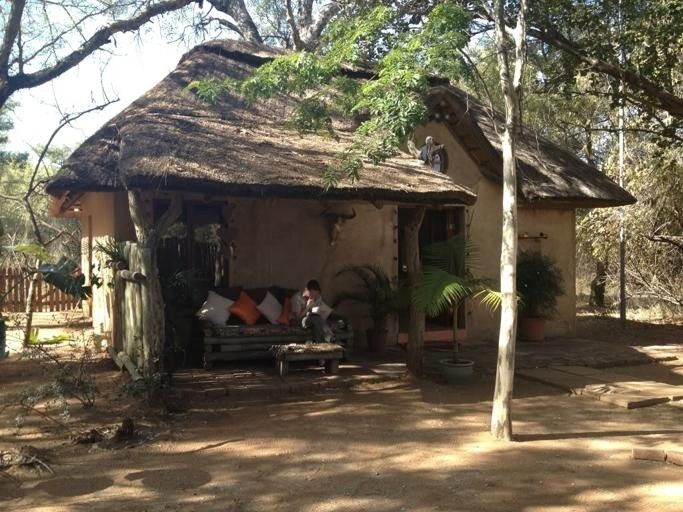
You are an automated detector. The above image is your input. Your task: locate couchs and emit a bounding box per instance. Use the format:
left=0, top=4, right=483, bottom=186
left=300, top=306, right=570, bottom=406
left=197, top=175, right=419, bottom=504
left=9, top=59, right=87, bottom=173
left=195, top=287, right=354, bottom=371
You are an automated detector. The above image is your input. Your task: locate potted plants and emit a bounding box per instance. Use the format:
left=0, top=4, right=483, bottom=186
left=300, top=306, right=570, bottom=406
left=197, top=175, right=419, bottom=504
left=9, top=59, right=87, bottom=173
left=515, top=244, right=564, bottom=342
left=331, top=264, right=407, bottom=352
left=409, top=232, right=525, bottom=385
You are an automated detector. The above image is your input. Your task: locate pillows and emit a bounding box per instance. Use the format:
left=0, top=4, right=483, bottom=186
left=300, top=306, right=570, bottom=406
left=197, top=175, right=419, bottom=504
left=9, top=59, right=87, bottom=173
left=196, top=291, right=335, bottom=325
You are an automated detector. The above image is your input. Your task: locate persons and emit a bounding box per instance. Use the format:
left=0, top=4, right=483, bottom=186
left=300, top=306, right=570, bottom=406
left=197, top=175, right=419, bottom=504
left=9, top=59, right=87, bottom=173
left=289, top=279, right=337, bottom=344
